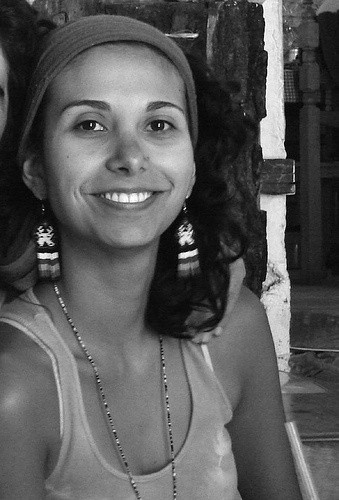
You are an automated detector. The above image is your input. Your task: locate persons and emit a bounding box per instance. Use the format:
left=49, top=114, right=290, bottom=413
left=0, top=1, right=48, bottom=307
left=0, top=15, right=303, bottom=500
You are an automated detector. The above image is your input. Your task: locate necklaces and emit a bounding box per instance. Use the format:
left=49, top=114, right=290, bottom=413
left=53, top=281, right=177, bottom=500
